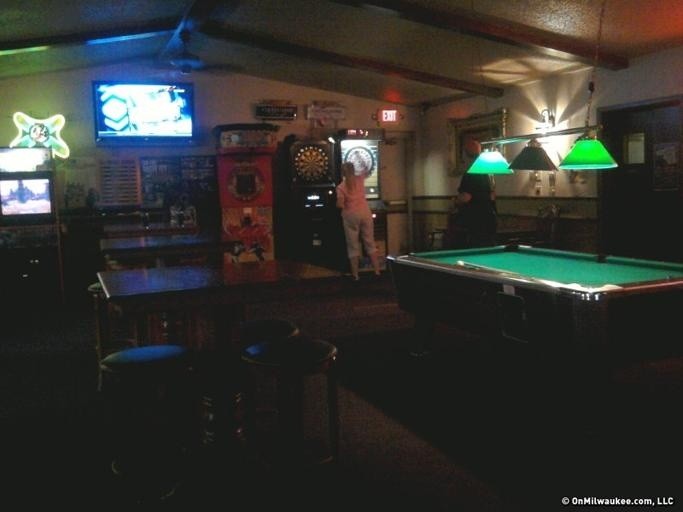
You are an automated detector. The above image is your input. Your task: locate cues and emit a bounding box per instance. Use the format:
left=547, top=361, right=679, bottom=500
left=465, top=260, right=520, bottom=275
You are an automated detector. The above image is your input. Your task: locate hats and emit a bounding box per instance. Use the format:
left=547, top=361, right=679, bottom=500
left=462, top=139, right=481, bottom=154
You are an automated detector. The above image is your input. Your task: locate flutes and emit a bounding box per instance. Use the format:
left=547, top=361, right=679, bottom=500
left=457, top=260, right=464, bottom=267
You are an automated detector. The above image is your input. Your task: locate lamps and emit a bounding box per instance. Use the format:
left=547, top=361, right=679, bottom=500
left=465, top=2, right=618, bottom=179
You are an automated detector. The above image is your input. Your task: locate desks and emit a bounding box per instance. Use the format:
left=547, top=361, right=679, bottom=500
left=384, top=244, right=683, bottom=410
left=95, top=222, right=353, bottom=465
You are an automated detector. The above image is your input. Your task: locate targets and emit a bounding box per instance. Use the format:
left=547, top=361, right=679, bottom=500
left=296, top=147, right=329, bottom=181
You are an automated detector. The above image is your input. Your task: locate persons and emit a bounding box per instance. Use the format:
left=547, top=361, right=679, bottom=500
left=170, top=192, right=197, bottom=228
left=453, top=141, right=497, bottom=249
left=336, top=151, right=381, bottom=281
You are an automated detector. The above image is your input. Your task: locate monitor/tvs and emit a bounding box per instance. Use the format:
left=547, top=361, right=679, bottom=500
left=0, top=171, right=56, bottom=225
left=91, top=79, right=197, bottom=147
